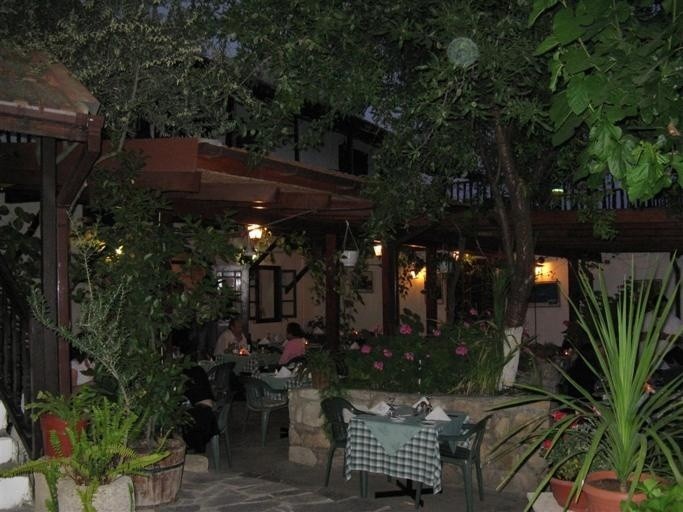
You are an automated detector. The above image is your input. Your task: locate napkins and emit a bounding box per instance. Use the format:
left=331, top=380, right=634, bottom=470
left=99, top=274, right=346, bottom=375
left=410, top=397, right=431, bottom=411
left=426, top=405, right=451, bottom=422
left=368, top=401, right=392, bottom=414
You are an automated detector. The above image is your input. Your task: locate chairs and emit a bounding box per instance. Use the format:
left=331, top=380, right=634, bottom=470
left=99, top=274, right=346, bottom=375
left=241, top=377, right=287, bottom=446
left=189, top=394, right=235, bottom=475
left=415, top=414, right=494, bottom=511
left=321, top=398, right=375, bottom=491
left=159, top=339, right=313, bottom=407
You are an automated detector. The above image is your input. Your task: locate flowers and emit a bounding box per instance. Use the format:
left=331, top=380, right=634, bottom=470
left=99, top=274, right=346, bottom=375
left=539, top=405, right=609, bottom=481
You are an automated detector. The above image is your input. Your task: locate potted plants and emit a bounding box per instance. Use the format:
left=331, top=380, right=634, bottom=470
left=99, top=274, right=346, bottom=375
left=308, top=245, right=371, bottom=340
left=0, top=386, right=174, bottom=512
left=64, top=124, right=247, bottom=508
left=483, top=243, right=683, bottom=512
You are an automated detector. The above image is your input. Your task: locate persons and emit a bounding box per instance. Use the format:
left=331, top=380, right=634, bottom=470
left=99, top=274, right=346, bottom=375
left=69, top=331, right=96, bottom=394
left=641, top=346, right=683, bottom=394
left=276, top=320, right=307, bottom=366
left=553, top=336, right=600, bottom=400
left=637, top=292, right=683, bottom=371
left=173, top=336, right=220, bottom=454
left=210, top=317, right=249, bottom=402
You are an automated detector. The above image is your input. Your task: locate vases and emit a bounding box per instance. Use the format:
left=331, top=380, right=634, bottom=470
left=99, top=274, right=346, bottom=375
left=545, top=477, right=589, bottom=512
left=37, top=412, right=90, bottom=462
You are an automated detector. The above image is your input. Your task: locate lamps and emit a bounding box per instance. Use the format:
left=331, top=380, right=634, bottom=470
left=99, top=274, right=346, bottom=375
left=372, top=239, right=383, bottom=262
left=409, top=269, right=417, bottom=279
left=246, top=224, right=263, bottom=242
